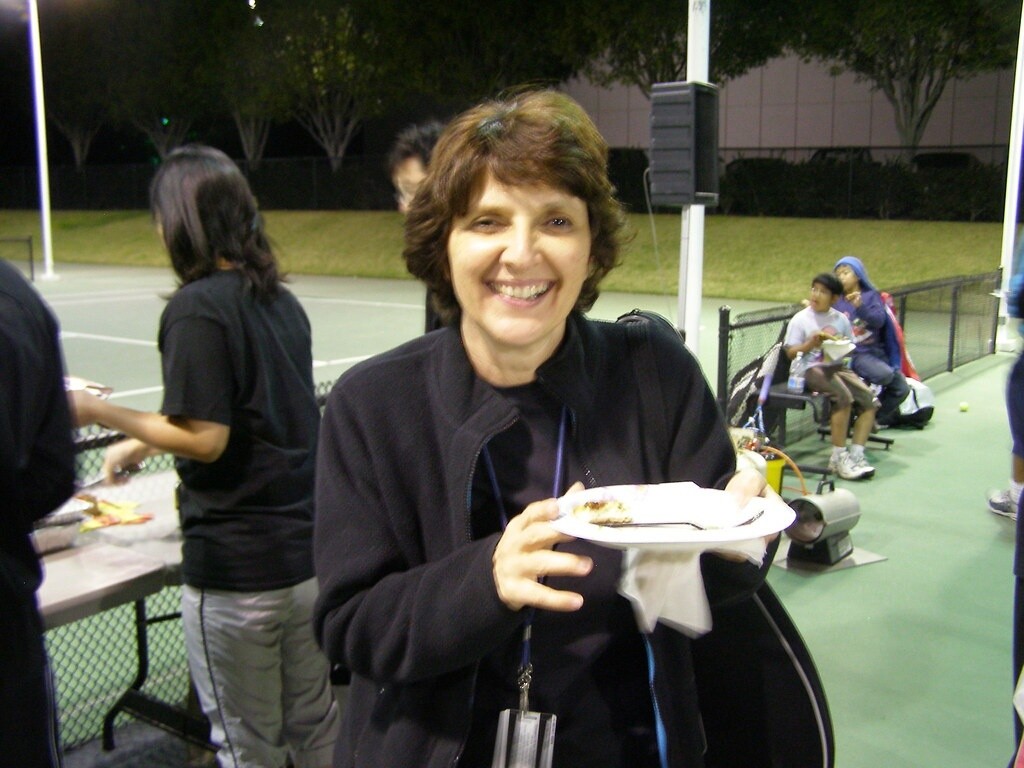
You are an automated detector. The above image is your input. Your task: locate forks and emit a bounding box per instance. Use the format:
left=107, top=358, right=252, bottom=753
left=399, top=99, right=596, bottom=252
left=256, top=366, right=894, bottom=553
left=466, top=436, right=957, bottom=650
left=590, top=509, right=765, bottom=530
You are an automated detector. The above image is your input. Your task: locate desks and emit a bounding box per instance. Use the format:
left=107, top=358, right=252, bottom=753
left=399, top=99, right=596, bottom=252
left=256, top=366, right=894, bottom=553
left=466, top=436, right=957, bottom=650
left=32, top=472, right=222, bottom=752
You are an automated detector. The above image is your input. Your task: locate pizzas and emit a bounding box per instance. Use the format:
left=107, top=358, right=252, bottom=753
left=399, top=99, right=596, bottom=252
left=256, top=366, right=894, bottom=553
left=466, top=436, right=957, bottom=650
left=819, top=332, right=837, bottom=341
left=573, top=499, right=633, bottom=523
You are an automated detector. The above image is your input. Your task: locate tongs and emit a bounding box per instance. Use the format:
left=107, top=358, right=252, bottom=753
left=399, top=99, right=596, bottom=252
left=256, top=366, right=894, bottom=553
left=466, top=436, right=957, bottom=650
left=74, top=461, right=147, bottom=494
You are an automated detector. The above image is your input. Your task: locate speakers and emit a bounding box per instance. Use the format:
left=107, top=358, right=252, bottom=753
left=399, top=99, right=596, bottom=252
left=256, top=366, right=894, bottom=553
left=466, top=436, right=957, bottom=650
left=647, top=79, right=722, bottom=207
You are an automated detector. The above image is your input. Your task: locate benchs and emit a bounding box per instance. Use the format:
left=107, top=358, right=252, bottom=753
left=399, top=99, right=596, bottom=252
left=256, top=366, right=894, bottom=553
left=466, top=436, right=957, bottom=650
left=753, top=378, right=896, bottom=479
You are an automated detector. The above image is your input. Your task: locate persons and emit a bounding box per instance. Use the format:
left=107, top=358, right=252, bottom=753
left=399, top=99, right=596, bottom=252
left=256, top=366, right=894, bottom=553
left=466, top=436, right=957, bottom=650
left=782, top=273, right=883, bottom=479
left=987, top=231, right=1024, bottom=520
left=64, top=146, right=340, bottom=768
left=311, top=88, right=781, bottom=768
left=828, top=255, right=910, bottom=436
left=0, top=255, right=80, bottom=768
left=390, top=122, right=452, bottom=338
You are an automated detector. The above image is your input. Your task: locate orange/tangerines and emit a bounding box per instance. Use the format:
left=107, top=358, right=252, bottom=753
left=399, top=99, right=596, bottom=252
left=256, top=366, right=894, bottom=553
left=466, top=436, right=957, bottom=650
left=959, top=402, right=968, bottom=411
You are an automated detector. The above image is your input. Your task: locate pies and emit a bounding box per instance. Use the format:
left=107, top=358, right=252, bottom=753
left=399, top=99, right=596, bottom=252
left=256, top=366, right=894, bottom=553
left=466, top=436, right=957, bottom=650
left=78, top=498, right=151, bottom=532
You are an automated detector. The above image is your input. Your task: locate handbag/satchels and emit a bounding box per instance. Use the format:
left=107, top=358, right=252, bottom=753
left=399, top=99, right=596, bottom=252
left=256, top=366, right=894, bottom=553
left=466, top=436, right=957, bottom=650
left=627, top=319, right=836, bottom=768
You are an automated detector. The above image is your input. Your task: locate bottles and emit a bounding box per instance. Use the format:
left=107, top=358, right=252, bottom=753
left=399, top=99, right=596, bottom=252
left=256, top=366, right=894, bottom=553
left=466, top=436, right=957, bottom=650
left=787, top=351, right=805, bottom=395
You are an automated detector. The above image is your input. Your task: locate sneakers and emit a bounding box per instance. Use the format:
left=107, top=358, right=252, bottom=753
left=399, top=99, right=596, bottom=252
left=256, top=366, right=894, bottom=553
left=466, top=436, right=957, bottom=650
left=855, top=456, right=875, bottom=477
left=989, top=490, right=1018, bottom=521
left=827, top=451, right=865, bottom=480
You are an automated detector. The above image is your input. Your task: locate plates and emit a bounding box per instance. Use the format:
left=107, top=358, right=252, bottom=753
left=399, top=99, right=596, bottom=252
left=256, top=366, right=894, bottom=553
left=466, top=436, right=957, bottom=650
left=550, top=485, right=796, bottom=548
left=64, top=376, right=108, bottom=400
